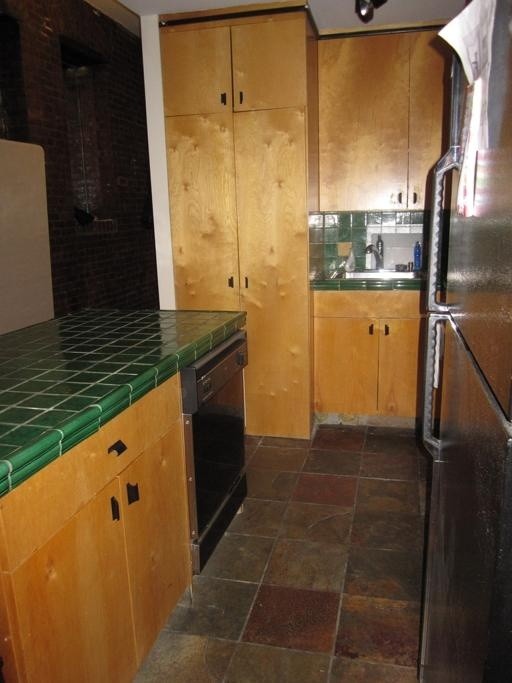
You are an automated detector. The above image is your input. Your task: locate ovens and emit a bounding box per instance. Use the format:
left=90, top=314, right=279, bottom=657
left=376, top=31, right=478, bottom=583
left=182, top=328, right=248, bottom=572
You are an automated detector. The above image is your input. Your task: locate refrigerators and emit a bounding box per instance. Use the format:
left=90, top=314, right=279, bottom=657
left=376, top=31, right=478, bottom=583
left=418, top=1, right=512, bottom=681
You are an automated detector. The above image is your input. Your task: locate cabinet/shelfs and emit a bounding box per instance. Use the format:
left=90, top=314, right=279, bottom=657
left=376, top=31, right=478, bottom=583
left=319, top=19, right=456, bottom=213
left=159, top=0, right=319, bottom=441
left=315, top=291, right=443, bottom=433
left=0, top=312, right=249, bottom=683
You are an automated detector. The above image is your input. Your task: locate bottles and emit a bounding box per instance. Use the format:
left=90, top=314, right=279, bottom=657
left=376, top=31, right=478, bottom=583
left=414, top=241, right=420, bottom=271
left=377, top=234, right=383, bottom=269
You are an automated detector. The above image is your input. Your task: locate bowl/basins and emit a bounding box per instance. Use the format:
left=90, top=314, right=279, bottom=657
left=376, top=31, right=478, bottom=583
left=396, top=265, right=407, bottom=272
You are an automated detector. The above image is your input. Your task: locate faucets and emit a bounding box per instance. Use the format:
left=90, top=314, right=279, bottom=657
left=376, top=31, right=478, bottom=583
left=366, top=234, right=384, bottom=271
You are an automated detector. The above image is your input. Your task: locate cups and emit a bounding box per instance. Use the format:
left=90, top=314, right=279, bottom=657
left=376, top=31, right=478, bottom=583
left=408, top=262, right=413, bottom=272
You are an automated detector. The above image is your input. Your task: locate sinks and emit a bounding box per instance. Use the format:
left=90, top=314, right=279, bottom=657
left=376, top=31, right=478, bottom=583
left=336, top=269, right=424, bottom=281
